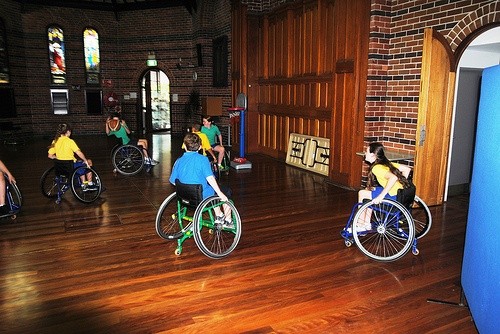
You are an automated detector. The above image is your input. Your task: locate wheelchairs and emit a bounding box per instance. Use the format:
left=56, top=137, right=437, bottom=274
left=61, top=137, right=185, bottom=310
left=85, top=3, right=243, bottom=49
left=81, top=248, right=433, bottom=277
left=108, top=134, right=144, bottom=176
left=0, top=174, right=22, bottom=220
left=199, top=143, right=231, bottom=176
left=40, top=159, right=102, bottom=204
left=339, top=185, right=432, bottom=263
left=155, top=179, right=242, bottom=260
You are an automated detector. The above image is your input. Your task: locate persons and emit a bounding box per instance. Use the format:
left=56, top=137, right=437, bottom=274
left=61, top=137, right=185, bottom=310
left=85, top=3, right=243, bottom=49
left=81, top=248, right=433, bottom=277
left=0, top=159, right=16, bottom=215
left=201, top=115, right=225, bottom=170
left=342, top=142, right=411, bottom=237
left=176, top=124, right=217, bottom=164
left=169, top=133, right=238, bottom=230
left=106, top=112, right=160, bottom=166
left=48, top=122, right=98, bottom=191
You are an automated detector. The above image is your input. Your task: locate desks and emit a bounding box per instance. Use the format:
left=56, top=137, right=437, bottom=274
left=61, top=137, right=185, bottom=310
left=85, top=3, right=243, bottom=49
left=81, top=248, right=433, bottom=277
left=356, top=152, right=413, bottom=162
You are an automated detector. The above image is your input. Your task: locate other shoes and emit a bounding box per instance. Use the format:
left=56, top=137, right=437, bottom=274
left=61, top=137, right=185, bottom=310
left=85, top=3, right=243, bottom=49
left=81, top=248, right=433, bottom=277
left=213, top=164, right=224, bottom=171
left=81, top=181, right=97, bottom=191
left=143, top=157, right=159, bottom=165
left=0, top=205, right=11, bottom=216
left=344, top=224, right=368, bottom=236
left=214, top=215, right=235, bottom=229
